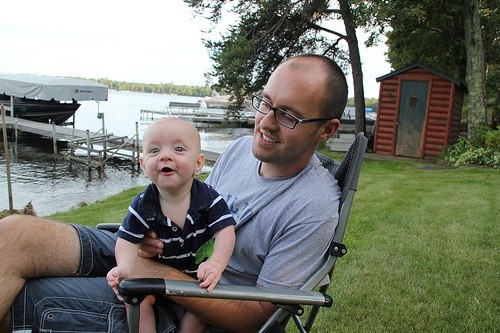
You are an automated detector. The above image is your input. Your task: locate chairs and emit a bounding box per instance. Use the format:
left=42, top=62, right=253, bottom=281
left=96, top=132, right=368, bottom=333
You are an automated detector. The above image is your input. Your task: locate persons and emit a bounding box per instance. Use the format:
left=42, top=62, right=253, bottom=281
left=107, top=118, right=237, bottom=333
left=0, top=54, right=348, bottom=333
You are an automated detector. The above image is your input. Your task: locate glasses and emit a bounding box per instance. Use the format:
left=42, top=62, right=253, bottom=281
left=251, top=94, right=331, bottom=130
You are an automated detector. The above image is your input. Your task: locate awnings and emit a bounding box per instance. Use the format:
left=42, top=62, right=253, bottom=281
left=0, top=74, right=108, bottom=101
